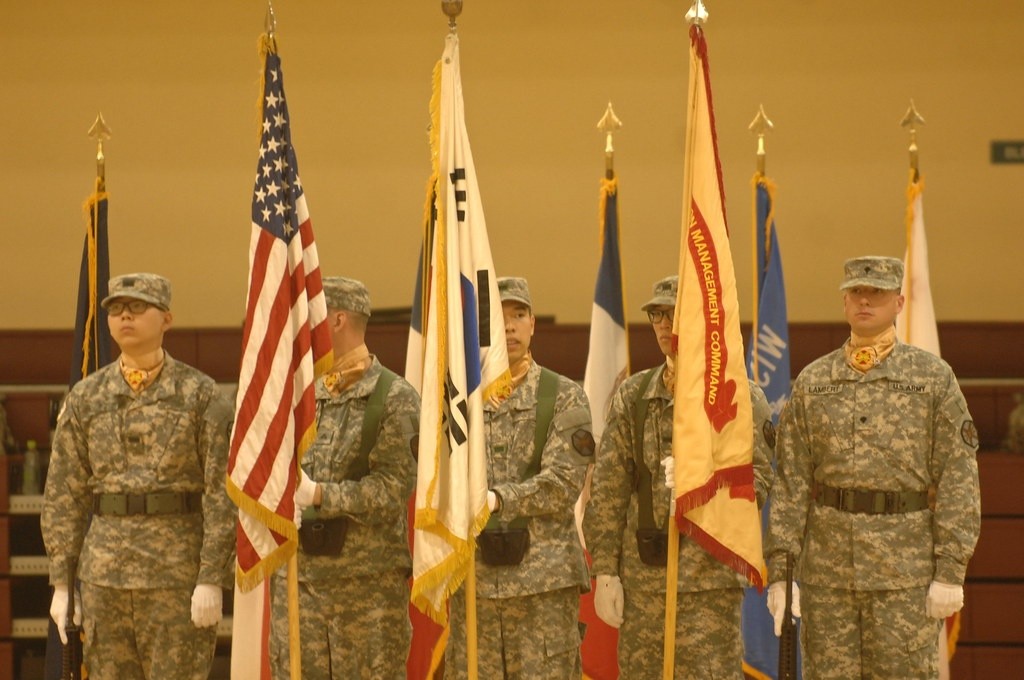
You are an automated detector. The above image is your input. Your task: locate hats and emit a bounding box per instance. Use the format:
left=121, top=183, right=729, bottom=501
left=498, top=278, right=532, bottom=307
left=840, top=257, right=904, bottom=290
left=641, top=276, right=678, bottom=311
left=323, top=278, right=372, bottom=317
left=101, top=273, right=171, bottom=311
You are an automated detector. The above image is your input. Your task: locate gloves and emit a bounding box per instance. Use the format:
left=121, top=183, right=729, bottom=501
left=660, top=454, right=674, bottom=488
left=767, top=581, right=802, bottom=637
left=292, top=504, right=302, bottom=531
left=594, top=574, right=624, bottom=629
left=50, top=583, right=81, bottom=645
left=926, top=580, right=964, bottom=618
left=293, top=469, right=316, bottom=507
left=191, top=584, right=223, bottom=628
left=487, top=490, right=496, bottom=512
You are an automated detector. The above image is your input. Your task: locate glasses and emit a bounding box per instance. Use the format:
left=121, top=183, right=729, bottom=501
left=105, top=300, right=151, bottom=314
left=647, top=309, right=674, bottom=323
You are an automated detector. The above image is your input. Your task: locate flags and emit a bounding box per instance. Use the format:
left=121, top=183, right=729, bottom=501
left=402, top=34, right=511, bottom=680
left=226, top=46, right=337, bottom=595
left=672, top=24, right=767, bottom=596
left=737, top=178, right=790, bottom=680
left=893, top=169, right=963, bottom=680
left=578, top=176, right=629, bottom=680
left=45, top=193, right=111, bottom=680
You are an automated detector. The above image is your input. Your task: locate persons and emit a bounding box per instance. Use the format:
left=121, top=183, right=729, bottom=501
left=765, top=255, right=980, bottom=680
left=581, top=273, right=776, bottom=680
left=267, top=277, right=421, bottom=680
left=40, top=271, right=237, bottom=680
left=443, top=276, right=595, bottom=680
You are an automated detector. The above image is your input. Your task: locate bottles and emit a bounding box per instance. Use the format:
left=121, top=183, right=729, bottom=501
left=22, top=399, right=61, bottom=495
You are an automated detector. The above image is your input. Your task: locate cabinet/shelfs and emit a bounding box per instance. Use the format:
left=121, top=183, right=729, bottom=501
left=0, top=383, right=240, bottom=639
